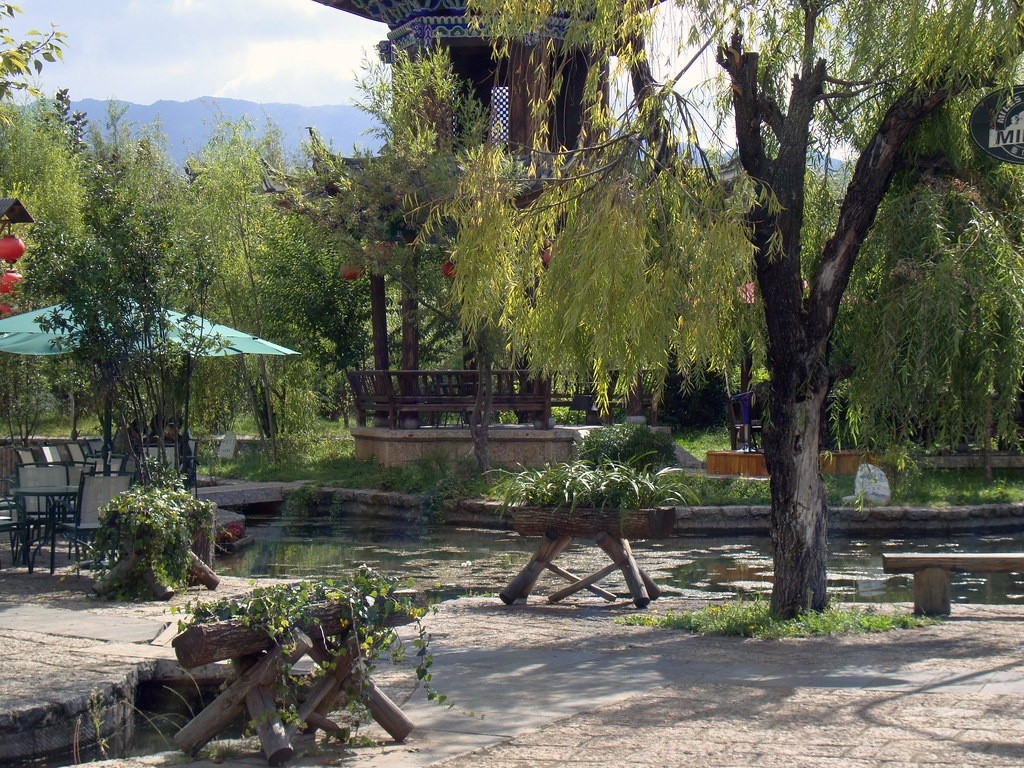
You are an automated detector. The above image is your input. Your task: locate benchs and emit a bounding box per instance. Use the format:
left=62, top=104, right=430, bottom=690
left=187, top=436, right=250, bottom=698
left=347, top=368, right=396, bottom=431
left=548, top=369, right=665, bottom=418
left=388, top=366, right=546, bottom=424
left=883, top=552, right=1024, bottom=617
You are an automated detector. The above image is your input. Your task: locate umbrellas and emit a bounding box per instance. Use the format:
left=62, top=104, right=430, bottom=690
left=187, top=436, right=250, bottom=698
left=0, top=298, right=303, bottom=478
left=689, top=276, right=873, bottom=306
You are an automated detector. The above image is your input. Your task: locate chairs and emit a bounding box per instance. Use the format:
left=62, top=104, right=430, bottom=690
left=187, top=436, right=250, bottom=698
left=1, top=438, right=202, bottom=578
left=734, top=425, right=763, bottom=452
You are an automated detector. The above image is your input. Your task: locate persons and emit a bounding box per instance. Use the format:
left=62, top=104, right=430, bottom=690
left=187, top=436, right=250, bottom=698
left=142, top=414, right=176, bottom=443
left=112, top=418, right=148, bottom=460
left=164, top=415, right=194, bottom=481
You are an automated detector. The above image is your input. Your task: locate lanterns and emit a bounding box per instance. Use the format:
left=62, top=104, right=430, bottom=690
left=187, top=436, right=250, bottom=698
left=0, top=235, right=26, bottom=264
left=340, top=260, right=364, bottom=280
left=369, top=241, right=394, bottom=261
left=442, top=260, right=457, bottom=278
left=0, top=303, right=19, bottom=315
left=0, top=268, right=23, bottom=294
